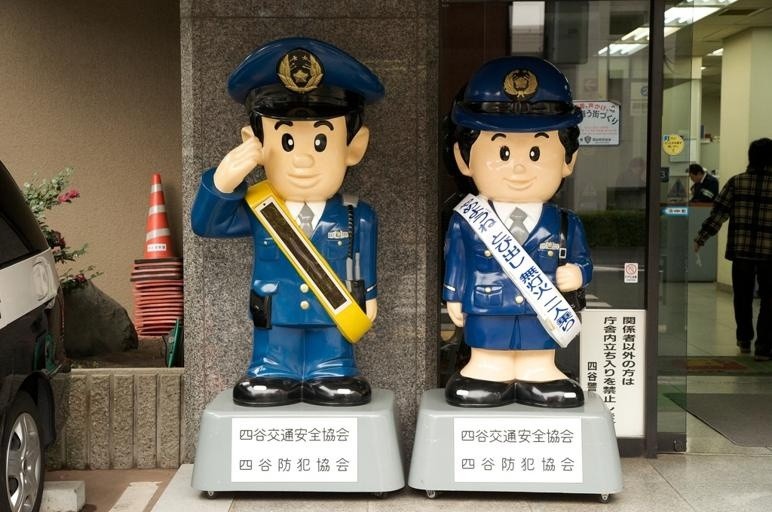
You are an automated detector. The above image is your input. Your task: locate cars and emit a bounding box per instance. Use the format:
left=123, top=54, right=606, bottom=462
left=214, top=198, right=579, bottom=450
left=0, top=159, right=65, bottom=512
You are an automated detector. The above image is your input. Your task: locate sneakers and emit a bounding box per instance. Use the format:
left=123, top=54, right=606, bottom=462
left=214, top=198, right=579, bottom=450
left=754, top=348, right=772, bottom=361
left=737, top=340, right=751, bottom=354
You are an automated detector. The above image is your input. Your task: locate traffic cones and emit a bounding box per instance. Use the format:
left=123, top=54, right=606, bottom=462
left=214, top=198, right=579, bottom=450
left=129, top=173, right=183, bottom=337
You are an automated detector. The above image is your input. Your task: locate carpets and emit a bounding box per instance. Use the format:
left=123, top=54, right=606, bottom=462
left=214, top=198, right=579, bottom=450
left=662, top=392, right=772, bottom=448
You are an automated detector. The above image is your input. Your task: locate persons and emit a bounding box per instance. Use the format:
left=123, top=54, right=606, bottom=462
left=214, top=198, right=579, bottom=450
left=442, top=54, right=593, bottom=408
left=692, top=138, right=772, bottom=362
left=615, top=158, right=645, bottom=209
left=191, top=38, right=384, bottom=406
left=685, top=164, right=719, bottom=203
